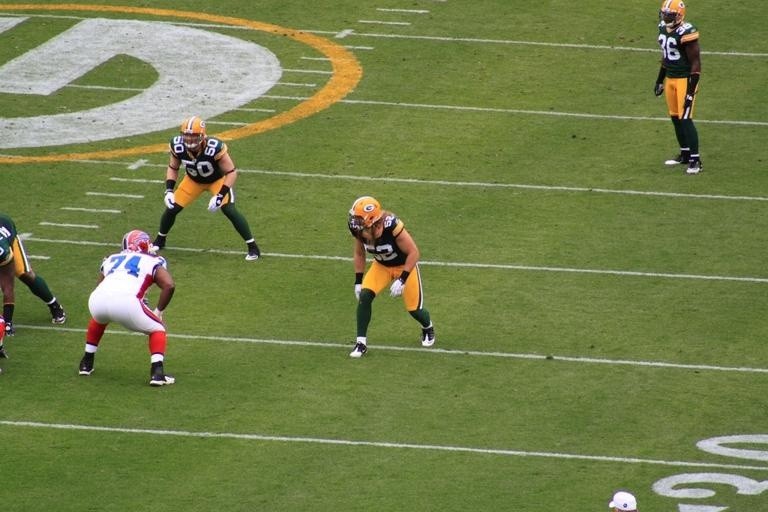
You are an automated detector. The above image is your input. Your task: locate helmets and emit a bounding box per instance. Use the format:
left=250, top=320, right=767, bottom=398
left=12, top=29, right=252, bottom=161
left=659, top=0, right=686, bottom=28
left=121, top=229, right=150, bottom=253
left=348, top=196, right=383, bottom=239
left=180, top=116, right=207, bottom=153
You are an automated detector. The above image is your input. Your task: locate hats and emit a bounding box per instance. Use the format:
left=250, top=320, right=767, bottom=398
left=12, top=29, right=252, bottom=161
left=609, top=491, right=637, bottom=511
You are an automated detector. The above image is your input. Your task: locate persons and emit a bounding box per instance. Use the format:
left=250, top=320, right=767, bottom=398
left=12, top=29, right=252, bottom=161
left=151, top=115, right=263, bottom=262
left=653, top=1, right=704, bottom=175
left=1, top=213, right=68, bottom=375
left=347, top=194, right=436, bottom=359
left=78, top=228, right=177, bottom=387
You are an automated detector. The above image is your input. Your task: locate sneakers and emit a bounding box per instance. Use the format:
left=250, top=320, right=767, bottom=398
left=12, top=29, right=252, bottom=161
left=79, top=361, right=94, bottom=376
left=150, top=242, right=165, bottom=255
left=421, top=327, right=435, bottom=347
left=5, top=320, right=16, bottom=336
left=686, top=157, right=703, bottom=175
left=349, top=341, right=368, bottom=358
left=665, top=153, right=690, bottom=165
left=51, top=304, right=66, bottom=325
left=150, top=375, right=176, bottom=386
left=245, top=250, right=260, bottom=260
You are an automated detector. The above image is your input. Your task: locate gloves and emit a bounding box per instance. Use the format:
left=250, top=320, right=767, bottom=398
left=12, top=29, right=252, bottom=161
left=654, top=81, right=663, bottom=96
left=208, top=194, right=223, bottom=212
left=354, top=284, right=362, bottom=301
left=390, top=279, right=405, bottom=298
left=164, top=192, right=175, bottom=210
left=153, top=307, right=164, bottom=320
left=684, top=94, right=693, bottom=109
